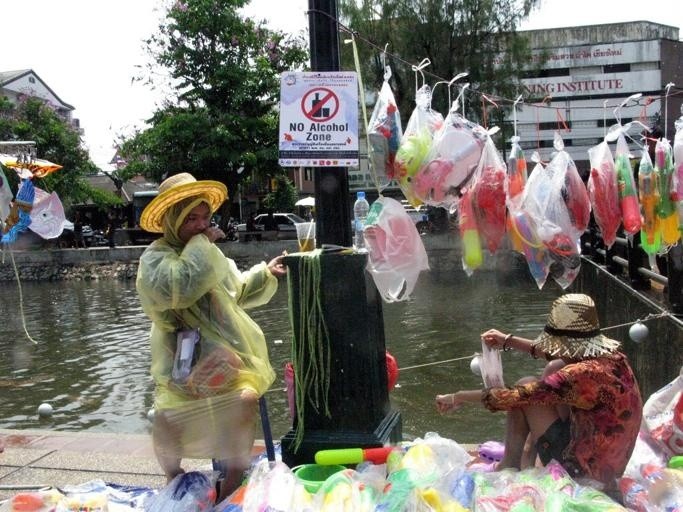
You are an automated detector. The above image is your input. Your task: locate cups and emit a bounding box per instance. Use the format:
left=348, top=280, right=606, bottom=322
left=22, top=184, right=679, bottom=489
left=295, top=222, right=315, bottom=253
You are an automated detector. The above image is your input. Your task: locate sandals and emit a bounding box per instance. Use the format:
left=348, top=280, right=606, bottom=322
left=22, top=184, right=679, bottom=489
left=470, top=441, right=505, bottom=473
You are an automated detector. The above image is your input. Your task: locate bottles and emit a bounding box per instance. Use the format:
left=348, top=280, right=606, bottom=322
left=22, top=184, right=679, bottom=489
left=352, top=191, right=370, bottom=253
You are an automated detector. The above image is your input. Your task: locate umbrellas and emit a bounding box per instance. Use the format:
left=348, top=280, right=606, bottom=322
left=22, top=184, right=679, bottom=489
left=294, top=196, right=316, bottom=208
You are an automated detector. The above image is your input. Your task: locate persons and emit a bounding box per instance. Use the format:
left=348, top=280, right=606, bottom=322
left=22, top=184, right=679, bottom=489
left=105, top=212, right=115, bottom=249
left=304, top=207, right=312, bottom=222
left=434, top=294, right=643, bottom=498
left=72, top=210, right=88, bottom=249
left=136, top=172, right=288, bottom=506
left=244, top=215, right=258, bottom=241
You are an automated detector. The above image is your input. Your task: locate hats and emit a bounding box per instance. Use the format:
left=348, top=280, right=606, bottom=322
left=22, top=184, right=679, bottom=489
left=530, top=293, right=621, bottom=359
left=140, top=172, right=229, bottom=233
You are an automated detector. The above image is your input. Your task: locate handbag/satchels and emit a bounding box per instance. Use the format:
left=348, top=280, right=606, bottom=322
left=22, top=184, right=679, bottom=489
left=186, top=346, right=243, bottom=399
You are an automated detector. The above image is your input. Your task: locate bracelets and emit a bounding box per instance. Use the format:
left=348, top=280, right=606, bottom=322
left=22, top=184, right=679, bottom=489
left=451, top=393, right=464, bottom=411
left=502, top=333, right=513, bottom=353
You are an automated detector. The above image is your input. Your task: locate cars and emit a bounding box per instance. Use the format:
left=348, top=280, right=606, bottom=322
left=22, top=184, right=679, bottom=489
left=237, top=213, right=308, bottom=240
left=59, top=219, right=94, bottom=248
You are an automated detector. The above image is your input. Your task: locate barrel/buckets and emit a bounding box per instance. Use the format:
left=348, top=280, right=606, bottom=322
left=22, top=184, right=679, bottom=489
left=427, top=206, right=449, bottom=233
left=290, top=462, right=346, bottom=492
